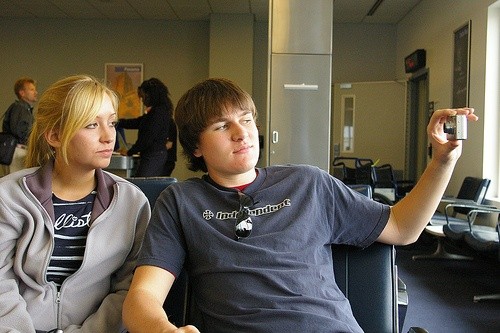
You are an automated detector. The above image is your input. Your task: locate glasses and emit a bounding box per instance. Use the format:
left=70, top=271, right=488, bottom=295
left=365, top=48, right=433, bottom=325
left=234, top=188, right=259, bottom=241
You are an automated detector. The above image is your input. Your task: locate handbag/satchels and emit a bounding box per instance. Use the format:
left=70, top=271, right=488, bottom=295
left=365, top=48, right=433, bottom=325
left=0, top=132, right=19, bottom=166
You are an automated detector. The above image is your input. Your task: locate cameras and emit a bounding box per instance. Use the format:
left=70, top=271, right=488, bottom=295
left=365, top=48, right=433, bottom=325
left=443, top=114, right=468, bottom=141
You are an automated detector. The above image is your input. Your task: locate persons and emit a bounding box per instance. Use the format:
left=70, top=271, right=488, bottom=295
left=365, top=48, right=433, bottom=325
left=121, top=77, right=478, bottom=333
left=2, top=78, right=37, bottom=175
left=0, top=76, right=150, bottom=333
left=116, top=78, right=177, bottom=176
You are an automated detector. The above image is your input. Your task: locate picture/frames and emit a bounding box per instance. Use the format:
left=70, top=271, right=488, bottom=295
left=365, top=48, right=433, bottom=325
left=103, top=63, right=143, bottom=119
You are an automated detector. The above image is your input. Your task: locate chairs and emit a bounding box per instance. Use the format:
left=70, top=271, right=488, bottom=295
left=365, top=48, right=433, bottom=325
left=126, top=157, right=500, bottom=333
